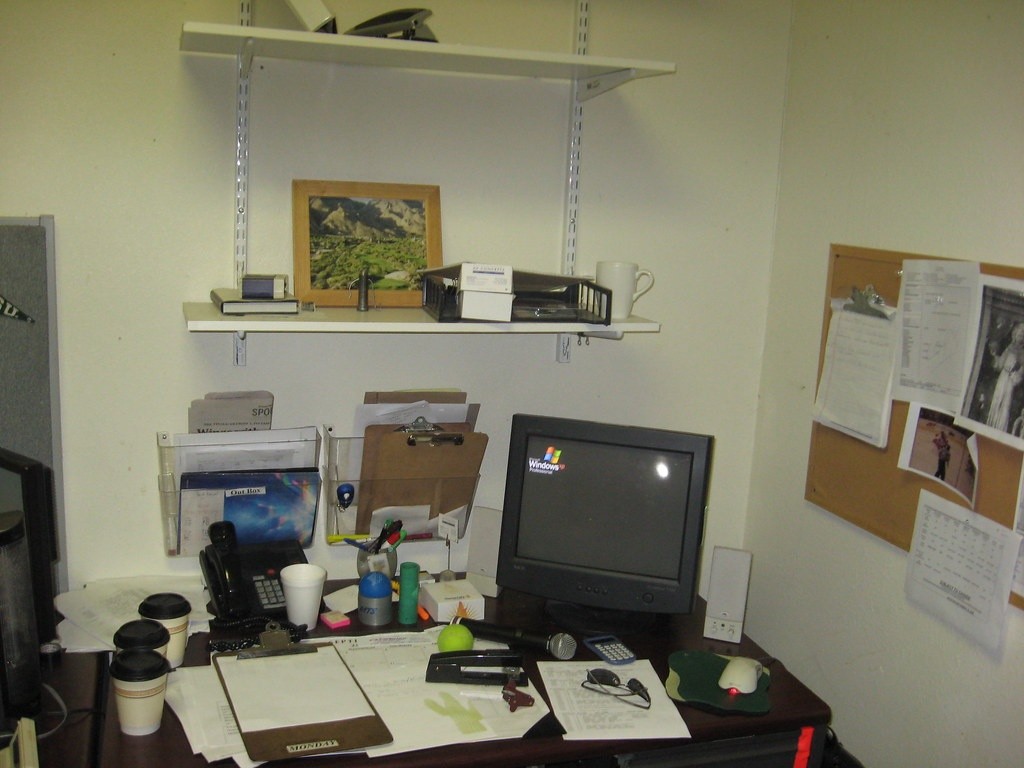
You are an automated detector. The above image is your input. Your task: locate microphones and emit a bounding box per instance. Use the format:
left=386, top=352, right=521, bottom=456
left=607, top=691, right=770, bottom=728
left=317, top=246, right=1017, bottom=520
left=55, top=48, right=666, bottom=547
left=450, top=616, right=577, bottom=660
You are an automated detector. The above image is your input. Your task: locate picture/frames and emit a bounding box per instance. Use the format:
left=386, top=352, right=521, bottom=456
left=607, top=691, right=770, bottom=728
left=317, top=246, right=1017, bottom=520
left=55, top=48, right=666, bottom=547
left=292, top=180, right=442, bottom=308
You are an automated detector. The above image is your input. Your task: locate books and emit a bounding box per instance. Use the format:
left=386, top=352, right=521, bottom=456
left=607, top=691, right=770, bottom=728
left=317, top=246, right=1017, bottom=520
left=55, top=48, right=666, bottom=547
left=209, top=288, right=299, bottom=315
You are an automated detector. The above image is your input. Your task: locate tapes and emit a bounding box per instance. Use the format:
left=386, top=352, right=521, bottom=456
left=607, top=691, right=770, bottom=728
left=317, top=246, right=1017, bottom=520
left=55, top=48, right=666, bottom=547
left=40, top=642, right=67, bottom=673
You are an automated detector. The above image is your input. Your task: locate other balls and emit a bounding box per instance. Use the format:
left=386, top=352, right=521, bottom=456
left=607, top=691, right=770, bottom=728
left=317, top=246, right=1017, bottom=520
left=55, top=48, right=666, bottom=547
left=438, top=623, right=474, bottom=652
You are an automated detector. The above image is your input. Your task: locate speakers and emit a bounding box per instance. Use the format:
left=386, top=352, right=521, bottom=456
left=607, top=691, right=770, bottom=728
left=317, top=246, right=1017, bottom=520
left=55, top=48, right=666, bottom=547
left=703, top=546, right=753, bottom=643
left=465, top=506, right=504, bottom=598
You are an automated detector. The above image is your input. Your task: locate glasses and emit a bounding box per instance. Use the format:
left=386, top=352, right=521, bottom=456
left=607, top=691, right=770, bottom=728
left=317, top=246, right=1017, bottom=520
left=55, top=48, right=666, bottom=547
left=581, top=668, right=652, bottom=708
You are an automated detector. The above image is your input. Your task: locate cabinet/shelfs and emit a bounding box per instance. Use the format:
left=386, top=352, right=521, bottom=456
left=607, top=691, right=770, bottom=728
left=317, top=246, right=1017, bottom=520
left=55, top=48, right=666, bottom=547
left=176, top=21, right=677, bottom=342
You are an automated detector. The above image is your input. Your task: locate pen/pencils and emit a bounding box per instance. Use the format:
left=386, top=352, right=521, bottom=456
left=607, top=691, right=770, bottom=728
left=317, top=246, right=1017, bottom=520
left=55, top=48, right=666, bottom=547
left=343, top=537, right=368, bottom=552
left=388, top=529, right=407, bottom=552
left=368, top=519, right=403, bottom=554
left=328, top=534, right=369, bottom=542
left=418, top=607, right=430, bottom=620
left=402, top=532, right=434, bottom=539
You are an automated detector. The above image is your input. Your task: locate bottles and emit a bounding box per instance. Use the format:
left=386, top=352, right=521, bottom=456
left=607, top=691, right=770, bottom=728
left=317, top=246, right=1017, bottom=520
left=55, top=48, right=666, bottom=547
left=357, top=571, right=392, bottom=626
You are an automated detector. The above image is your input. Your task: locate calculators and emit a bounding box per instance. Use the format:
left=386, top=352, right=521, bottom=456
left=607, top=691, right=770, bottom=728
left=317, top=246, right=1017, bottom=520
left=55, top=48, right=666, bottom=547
left=583, top=635, right=637, bottom=665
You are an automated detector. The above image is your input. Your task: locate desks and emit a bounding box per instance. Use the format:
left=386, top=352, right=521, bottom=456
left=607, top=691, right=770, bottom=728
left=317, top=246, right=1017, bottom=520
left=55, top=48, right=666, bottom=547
left=106, top=571, right=831, bottom=768
left=0, top=648, right=109, bottom=768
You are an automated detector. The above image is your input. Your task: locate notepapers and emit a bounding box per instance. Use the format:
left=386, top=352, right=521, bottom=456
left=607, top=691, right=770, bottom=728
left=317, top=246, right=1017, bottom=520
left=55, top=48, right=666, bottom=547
left=320, top=610, right=350, bottom=629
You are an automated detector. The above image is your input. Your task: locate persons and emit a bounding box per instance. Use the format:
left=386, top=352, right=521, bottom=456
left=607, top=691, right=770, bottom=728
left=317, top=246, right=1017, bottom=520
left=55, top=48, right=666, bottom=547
left=985, top=324, right=1024, bottom=432
left=933, top=431, right=950, bottom=481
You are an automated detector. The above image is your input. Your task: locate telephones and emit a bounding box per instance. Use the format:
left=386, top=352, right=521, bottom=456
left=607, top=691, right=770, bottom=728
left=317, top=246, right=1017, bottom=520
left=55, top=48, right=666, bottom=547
left=197, top=520, right=328, bottom=625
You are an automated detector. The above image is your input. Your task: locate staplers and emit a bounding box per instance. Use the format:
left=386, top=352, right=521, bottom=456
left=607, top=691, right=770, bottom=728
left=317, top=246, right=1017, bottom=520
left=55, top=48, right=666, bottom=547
left=426, top=649, right=529, bottom=687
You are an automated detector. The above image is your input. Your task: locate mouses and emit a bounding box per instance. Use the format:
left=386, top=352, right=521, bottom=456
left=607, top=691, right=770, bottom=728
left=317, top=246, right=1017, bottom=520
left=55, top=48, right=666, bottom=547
left=718, top=655, right=763, bottom=694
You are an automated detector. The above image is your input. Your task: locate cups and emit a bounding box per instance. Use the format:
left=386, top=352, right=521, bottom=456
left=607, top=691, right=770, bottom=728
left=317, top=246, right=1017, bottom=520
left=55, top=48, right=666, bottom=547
left=357, top=542, right=398, bottom=580
left=112, top=620, right=170, bottom=660
left=596, top=261, right=654, bottom=321
left=279, top=563, right=326, bottom=631
left=108, top=648, right=171, bottom=737
left=138, top=592, right=192, bottom=669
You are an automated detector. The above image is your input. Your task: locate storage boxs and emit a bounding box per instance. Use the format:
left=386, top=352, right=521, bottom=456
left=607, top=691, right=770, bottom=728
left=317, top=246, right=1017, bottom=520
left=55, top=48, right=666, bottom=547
left=417, top=580, right=486, bottom=624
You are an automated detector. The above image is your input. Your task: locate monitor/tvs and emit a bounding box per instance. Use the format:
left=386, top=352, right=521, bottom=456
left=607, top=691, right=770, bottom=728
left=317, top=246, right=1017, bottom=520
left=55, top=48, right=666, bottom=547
left=496, top=414, right=715, bottom=638
